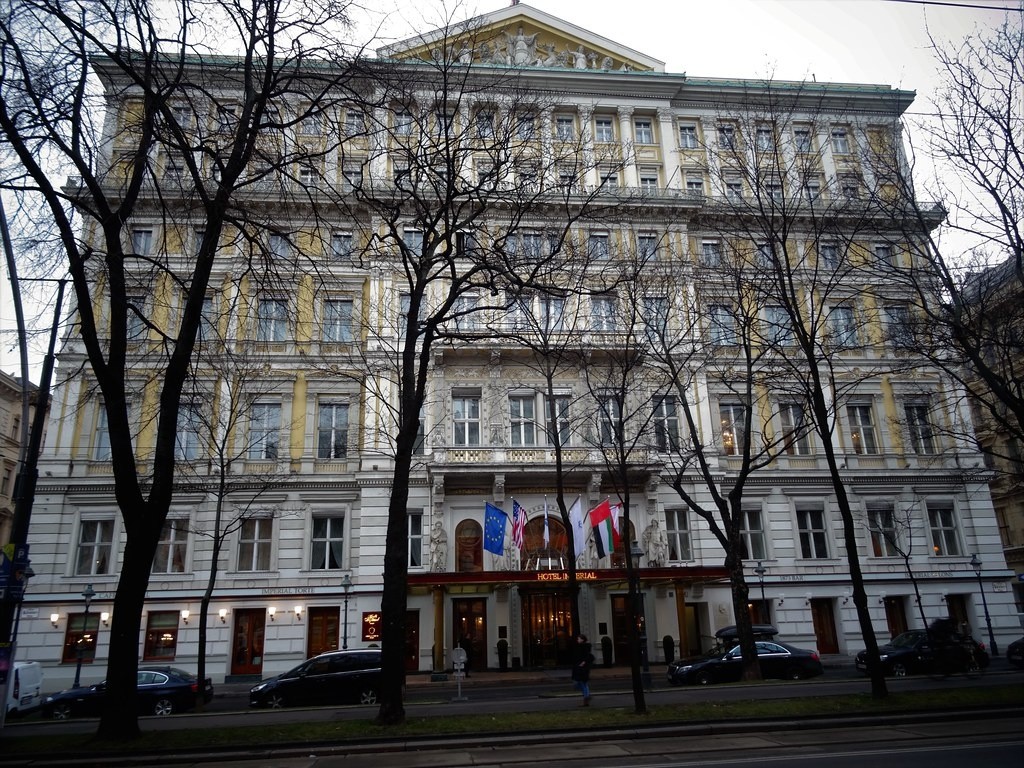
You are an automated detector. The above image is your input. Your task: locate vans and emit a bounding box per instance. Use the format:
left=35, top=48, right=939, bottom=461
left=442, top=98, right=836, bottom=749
left=5, top=661, right=42, bottom=722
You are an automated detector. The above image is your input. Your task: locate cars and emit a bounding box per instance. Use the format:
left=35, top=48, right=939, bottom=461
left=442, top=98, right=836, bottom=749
left=1005, top=636, right=1024, bottom=672
left=248, top=646, right=384, bottom=711
left=40, top=666, right=215, bottom=721
left=854, top=629, right=990, bottom=677
left=665, top=624, right=823, bottom=688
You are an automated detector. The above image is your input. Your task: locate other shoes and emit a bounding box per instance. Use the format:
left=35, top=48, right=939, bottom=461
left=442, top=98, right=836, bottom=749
left=584, top=698, right=589, bottom=706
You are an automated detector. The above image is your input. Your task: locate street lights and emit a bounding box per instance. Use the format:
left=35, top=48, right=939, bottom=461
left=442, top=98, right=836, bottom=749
left=970, top=552, right=1000, bottom=656
left=629, top=538, right=650, bottom=671
left=72, top=582, right=96, bottom=689
left=340, top=574, right=353, bottom=650
left=754, top=562, right=769, bottom=624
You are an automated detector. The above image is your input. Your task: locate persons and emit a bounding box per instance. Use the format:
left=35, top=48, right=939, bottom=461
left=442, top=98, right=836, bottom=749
left=643, top=518, right=668, bottom=567
left=500, top=26, right=541, bottom=67
left=569, top=634, right=595, bottom=707
left=452, top=40, right=482, bottom=63
left=566, top=42, right=588, bottom=69
left=431, top=521, right=447, bottom=570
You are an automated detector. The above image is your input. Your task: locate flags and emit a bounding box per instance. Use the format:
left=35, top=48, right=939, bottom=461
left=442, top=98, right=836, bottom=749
left=542, top=499, right=550, bottom=549
left=589, top=499, right=622, bottom=559
left=568, top=497, right=587, bottom=560
left=484, top=502, right=508, bottom=555
left=512, top=498, right=528, bottom=554
left=611, top=501, right=626, bottom=536
